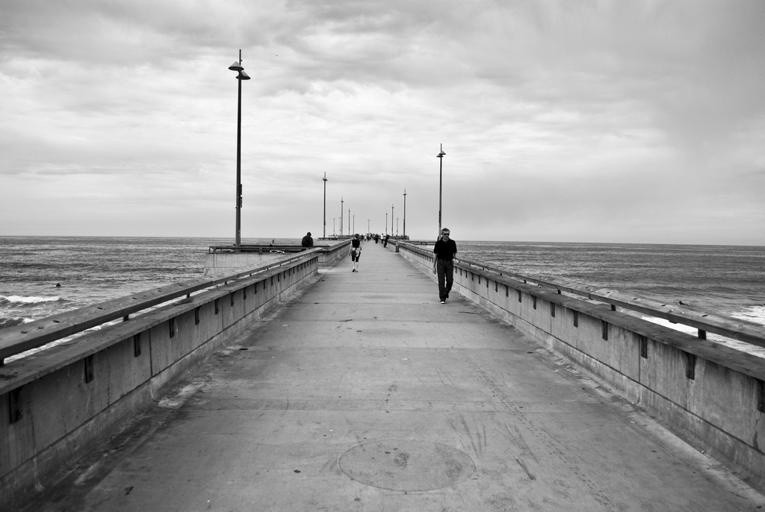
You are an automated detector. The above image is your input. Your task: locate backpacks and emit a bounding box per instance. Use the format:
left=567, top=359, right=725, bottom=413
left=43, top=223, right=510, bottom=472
left=351, top=238, right=360, bottom=248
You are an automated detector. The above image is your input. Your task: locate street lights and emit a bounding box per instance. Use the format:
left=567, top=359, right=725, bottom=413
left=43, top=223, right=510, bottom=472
left=352, top=214, right=354, bottom=233
left=385, top=212, right=388, bottom=236
left=391, top=204, right=393, bottom=237
left=340, top=196, right=344, bottom=235
left=322, top=172, right=329, bottom=238
left=436, top=142, right=446, bottom=240
left=348, top=208, right=350, bottom=235
left=228, top=48, right=251, bottom=244
left=403, top=188, right=406, bottom=236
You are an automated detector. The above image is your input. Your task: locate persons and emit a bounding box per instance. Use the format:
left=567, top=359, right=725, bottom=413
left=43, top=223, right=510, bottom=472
left=433, top=228, right=457, bottom=304
left=360, top=232, right=389, bottom=247
left=301, top=232, right=313, bottom=248
left=348, top=234, right=362, bottom=272
